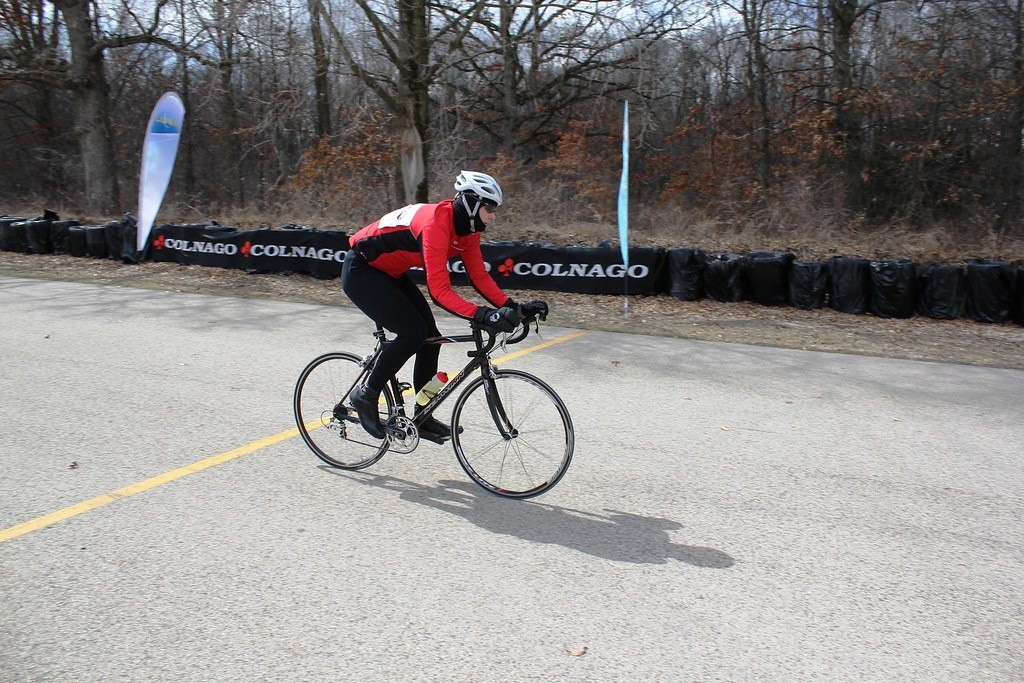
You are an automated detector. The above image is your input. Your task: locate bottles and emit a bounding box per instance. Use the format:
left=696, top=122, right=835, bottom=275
left=415, top=371, right=449, bottom=407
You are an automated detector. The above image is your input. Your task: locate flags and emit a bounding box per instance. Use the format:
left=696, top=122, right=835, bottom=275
left=618, top=103, right=629, bottom=269
left=140, top=96, right=186, bottom=250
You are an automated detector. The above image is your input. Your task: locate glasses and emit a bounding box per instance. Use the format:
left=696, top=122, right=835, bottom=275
left=463, top=193, right=496, bottom=213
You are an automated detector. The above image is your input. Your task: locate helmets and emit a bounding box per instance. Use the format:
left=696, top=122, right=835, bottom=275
left=453, top=170, right=503, bottom=206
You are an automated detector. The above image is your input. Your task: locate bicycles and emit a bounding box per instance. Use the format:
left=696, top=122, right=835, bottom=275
left=293, top=312, right=575, bottom=500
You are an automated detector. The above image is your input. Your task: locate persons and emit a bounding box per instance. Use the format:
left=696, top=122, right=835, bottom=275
left=341, top=170, right=549, bottom=440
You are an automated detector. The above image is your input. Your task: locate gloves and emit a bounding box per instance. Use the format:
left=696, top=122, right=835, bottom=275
left=473, top=306, right=520, bottom=333
left=502, top=298, right=549, bottom=321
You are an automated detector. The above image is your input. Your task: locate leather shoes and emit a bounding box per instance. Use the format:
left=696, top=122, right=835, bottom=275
left=412, top=406, right=463, bottom=436
left=349, top=384, right=386, bottom=439
